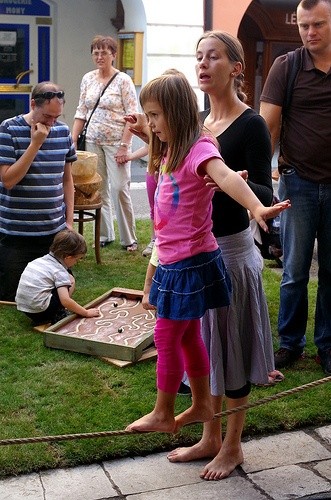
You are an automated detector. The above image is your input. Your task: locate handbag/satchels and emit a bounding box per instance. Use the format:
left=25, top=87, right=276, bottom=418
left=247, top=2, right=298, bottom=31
left=77, top=131, right=85, bottom=151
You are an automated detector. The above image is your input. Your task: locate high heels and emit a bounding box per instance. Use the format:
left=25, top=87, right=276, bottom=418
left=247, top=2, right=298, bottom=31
left=323, top=363, right=331, bottom=374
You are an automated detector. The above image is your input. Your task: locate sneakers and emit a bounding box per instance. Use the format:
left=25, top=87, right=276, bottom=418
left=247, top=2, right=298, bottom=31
left=143, top=240, right=155, bottom=257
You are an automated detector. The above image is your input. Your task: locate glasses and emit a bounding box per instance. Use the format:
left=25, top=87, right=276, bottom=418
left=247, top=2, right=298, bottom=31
left=92, top=52, right=112, bottom=56
left=32, top=92, right=65, bottom=99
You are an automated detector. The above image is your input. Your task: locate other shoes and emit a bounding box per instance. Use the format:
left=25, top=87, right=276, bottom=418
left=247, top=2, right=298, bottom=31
left=92, top=241, right=114, bottom=248
left=127, top=241, right=138, bottom=251
left=274, top=348, right=302, bottom=368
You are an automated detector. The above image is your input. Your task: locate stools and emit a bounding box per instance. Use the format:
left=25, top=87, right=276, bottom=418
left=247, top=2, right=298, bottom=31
left=73, top=202, right=103, bottom=264
